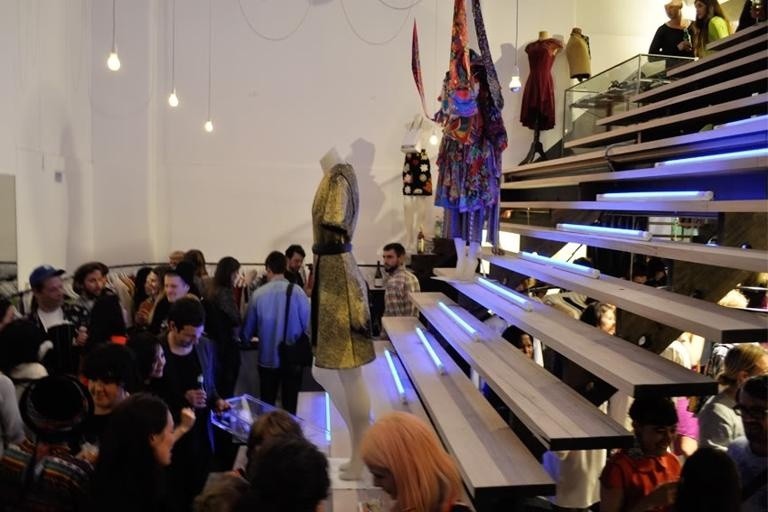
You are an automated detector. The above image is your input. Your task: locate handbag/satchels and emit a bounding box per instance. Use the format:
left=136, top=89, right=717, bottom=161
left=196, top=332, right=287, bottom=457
left=279, top=281, right=312, bottom=377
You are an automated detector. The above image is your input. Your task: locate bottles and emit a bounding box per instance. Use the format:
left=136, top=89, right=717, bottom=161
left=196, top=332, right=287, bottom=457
left=374, top=261, right=383, bottom=288
left=417, top=225, right=424, bottom=253
left=191, top=375, right=206, bottom=425
left=434, top=216, right=442, bottom=239
left=683, top=26, right=693, bottom=51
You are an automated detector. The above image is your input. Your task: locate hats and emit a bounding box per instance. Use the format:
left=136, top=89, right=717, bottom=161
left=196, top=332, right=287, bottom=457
left=85, top=345, right=140, bottom=382
left=28, top=266, right=66, bottom=287
left=22, top=373, right=96, bottom=429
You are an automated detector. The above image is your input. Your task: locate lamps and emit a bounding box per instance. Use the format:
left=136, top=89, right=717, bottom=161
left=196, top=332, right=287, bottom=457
left=507, top=0, right=523, bottom=92
left=205, top=0, right=214, bottom=132
left=597, top=191, right=713, bottom=202
left=415, top=326, right=447, bottom=375
left=474, top=276, right=533, bottom=312
left=655, top=148, right=768, bottom=170
left=556, top=223, right=652, bottom=243
left=430, top=0, right=437, bottom=145
left=325, top=391, right=331, bottom=442
left=517, top=251, right=600, bottom=279
left=714, top=114, right=768, bottom=129
left=107, top=0, right=120, bottom=71
left=169, top=0, right=179, bottom=107
left=438, top=301, right=483, bottom=342
left=383, top=351, right=409, bottom=406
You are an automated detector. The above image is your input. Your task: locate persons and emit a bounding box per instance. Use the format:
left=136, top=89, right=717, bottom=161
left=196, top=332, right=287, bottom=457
left=648, top=0, right=696, bottom=69
left=580, top=302, right=616, bottom=336
left=71, top=264, right=102, bottom=340
left=624, top=263, right=648, bottom=284
left=0, top=369, right=94, bottom=512
left=164, top=270, right=190, bottom=302
left=1, top=373, right=24, bottom=455
left=247, top=434, right=332, bottom=512
left=285, top=244, right=303, bottom=286
left=128, top=333, right=166, bottom=392
left=694, top=0, right=737, bottom=58
left=601, top=398, right=682, bottom=511
left=83, top=344, right=134, bottom=421
left=28, top=266, right=91, bottom=369
left=247, top=408, right=304, bottom=448
left=135, top=267, right=160, bottom=333
left=676, top=448, right=743, bottom=512
left=0, top=298, right=14, bottom=330
left=725, top=375, right=768, bottom=511
left=177, top=250, right=205, bottom=298
left=362, top=412, right=476, bottom=512
left=241, top=251, right=310, bottom=415
left=154, top=297, right=234, bottom=495
left=0, top=321, right=52, bottom=405
left=204, top=257, right=242, bottom=396
left=380, top=243, right=423, bottom=342
left=195, top=474, right=249, bottom=512
left=481, top=325, right=533, bottom=420
left=697, top=345, right=768, bottom=451
left=90, top=261, right=125, bottom=333
left=649, top=259, right=667, bottom=286
left=94, top=394, right=174, bottom=512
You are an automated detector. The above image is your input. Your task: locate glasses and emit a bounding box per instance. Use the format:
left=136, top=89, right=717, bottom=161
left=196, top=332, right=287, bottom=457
left=734, top=402, right=768, bottom=418
